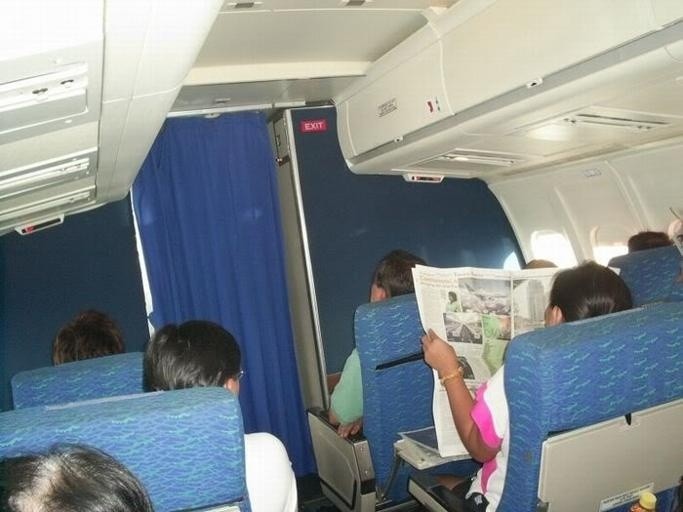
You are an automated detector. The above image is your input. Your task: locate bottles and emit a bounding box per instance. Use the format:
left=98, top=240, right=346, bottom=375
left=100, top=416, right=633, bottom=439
left=629, top=491, right=658, bottom=512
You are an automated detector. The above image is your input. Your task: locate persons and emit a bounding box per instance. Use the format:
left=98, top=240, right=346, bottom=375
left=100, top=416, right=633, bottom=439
left=50, top=310, right=121, bottom=362
left=444, top=291, right=459, bottom=312
left=417, top=262, right=631, bottom=512
left=142, top=319, right=299, bottom=512
left=320, top=247, right=425, bottom=441
left=0, top=441, right=154, bottom=511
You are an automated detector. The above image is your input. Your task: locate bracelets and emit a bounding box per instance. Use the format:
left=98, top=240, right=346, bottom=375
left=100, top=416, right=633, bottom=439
left=437, top=365, right=464, bottom=385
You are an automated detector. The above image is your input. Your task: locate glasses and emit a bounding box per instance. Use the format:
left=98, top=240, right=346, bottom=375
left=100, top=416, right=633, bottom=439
left=233, top=365, right=244, bottom=380
